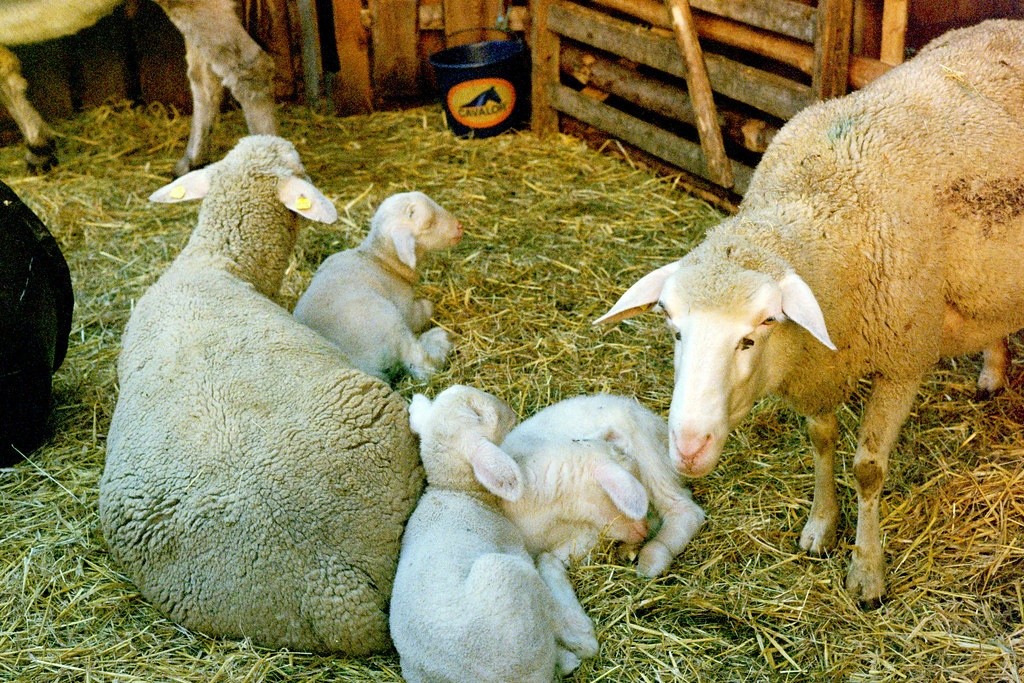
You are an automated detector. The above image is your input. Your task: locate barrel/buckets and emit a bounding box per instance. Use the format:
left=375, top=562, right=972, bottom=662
left=428, top=28, right=527, bottom=139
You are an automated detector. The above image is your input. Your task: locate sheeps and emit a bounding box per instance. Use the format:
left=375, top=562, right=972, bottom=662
left=388, top=382, right=707, bottom=683
left=0, top=1, right=279, bottom=179
left=97, top=134, right=428, bottom=655
left=291, top=190, right=464, bottom=388
left=589, top=17, right=1024, bottom=614
